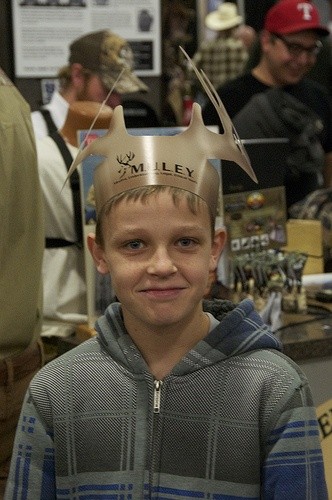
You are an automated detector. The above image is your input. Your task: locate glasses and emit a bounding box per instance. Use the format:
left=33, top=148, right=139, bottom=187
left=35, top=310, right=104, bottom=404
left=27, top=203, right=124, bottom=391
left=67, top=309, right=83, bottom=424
left=270, top=31, right=324, bottom=55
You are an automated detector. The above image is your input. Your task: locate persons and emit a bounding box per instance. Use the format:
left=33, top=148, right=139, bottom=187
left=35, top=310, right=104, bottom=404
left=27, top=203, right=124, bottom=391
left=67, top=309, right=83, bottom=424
left=0, top=65, right=48, bottom=500
left=161, top=1, right=332, bottom=231
left=30, top=29, right=149, bottom=343
left=2, top=151, right=330, bottom=500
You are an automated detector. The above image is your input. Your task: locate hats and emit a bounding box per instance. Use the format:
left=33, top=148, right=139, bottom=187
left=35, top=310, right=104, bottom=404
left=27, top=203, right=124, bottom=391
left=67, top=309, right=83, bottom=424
left=68, top=29, right=149, bottom=95
left=263, top=0, right=330, bottom=39
left=204, top=2, right=244, bottom=31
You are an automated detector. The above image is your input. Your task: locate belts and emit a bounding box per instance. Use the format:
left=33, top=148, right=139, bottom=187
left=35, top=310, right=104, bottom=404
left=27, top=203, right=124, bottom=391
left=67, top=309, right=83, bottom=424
left=0, top=339, right=42, bottom=383
